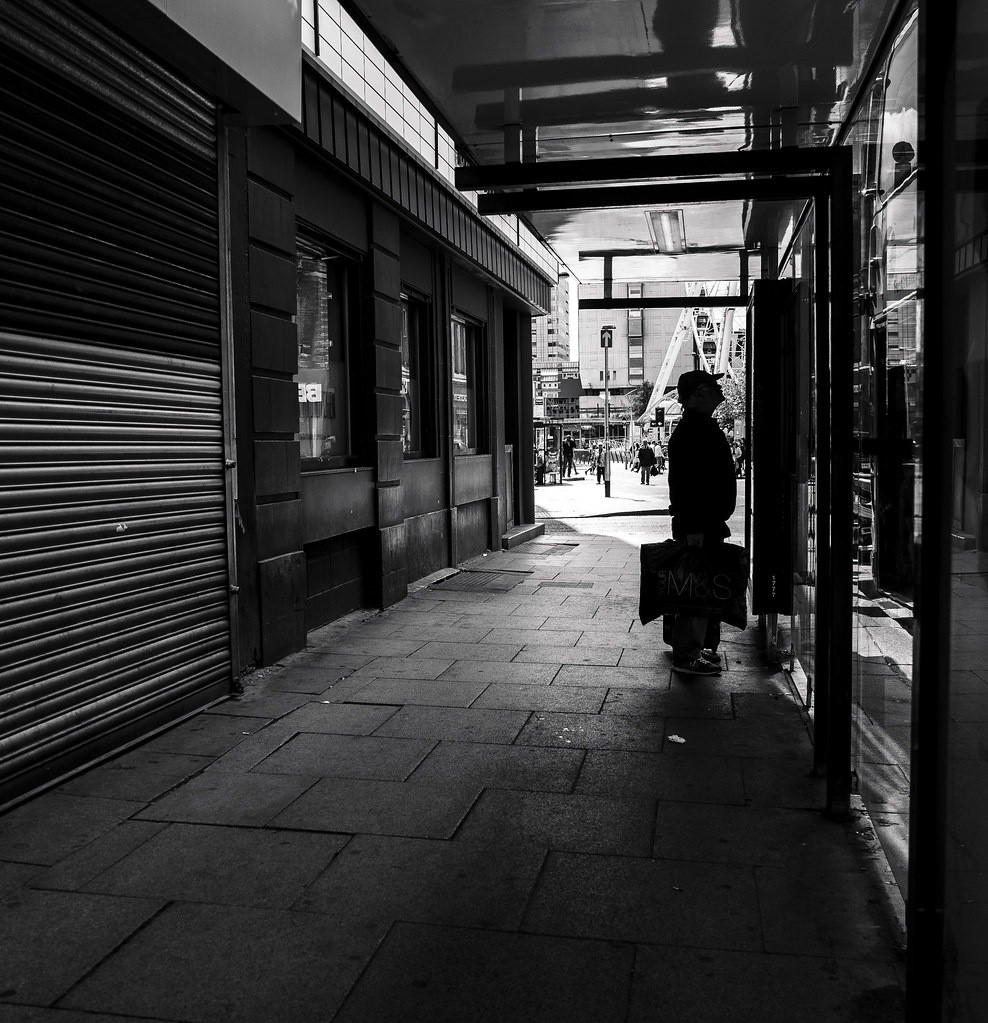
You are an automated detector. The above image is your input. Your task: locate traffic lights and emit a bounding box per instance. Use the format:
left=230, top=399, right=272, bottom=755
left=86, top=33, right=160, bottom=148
left=651, top=420, right=665, bottom=427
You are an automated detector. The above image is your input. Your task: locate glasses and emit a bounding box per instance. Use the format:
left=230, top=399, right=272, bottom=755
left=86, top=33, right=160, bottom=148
left=696, top=378, right=717, bottom=392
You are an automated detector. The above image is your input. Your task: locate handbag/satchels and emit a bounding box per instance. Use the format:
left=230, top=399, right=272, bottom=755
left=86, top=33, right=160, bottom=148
left=660, top=614, right=708, bottom=650
left=651, top=465, right=658, bottom=476
left=641, top=540, right=749, bottom=630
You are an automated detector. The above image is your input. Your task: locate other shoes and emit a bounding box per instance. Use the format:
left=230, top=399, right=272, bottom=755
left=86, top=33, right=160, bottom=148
left=646, top=483, right=649, bottom=485
left=641, top=482, right=644, bottom=483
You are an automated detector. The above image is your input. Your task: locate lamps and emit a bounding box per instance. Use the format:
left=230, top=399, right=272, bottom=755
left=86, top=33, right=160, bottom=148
left=644, top=209, right=687, bottom=256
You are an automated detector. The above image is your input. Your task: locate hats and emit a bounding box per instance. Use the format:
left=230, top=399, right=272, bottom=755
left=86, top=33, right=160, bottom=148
left=678, top=370, right=724, bottom=402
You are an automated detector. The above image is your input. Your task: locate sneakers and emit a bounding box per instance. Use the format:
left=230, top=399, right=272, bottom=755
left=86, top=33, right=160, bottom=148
left=701, top=650, right=721, bottom=664
left=671, top=656, right=722, bottom=674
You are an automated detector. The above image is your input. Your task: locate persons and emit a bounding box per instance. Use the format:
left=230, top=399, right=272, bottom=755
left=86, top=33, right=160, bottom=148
left=667, top=370, right=737, bottom=675
left=595, top=441, right=606, bottom=484
left=563, top=434, right=575, bottom=478
left=630, top=441, right=667, bottom=474
left=729, top=437, right=747, bottom=479
left=639, top=439, right=656, bottom=485
left=585, top=451, right=597, bottom=475
